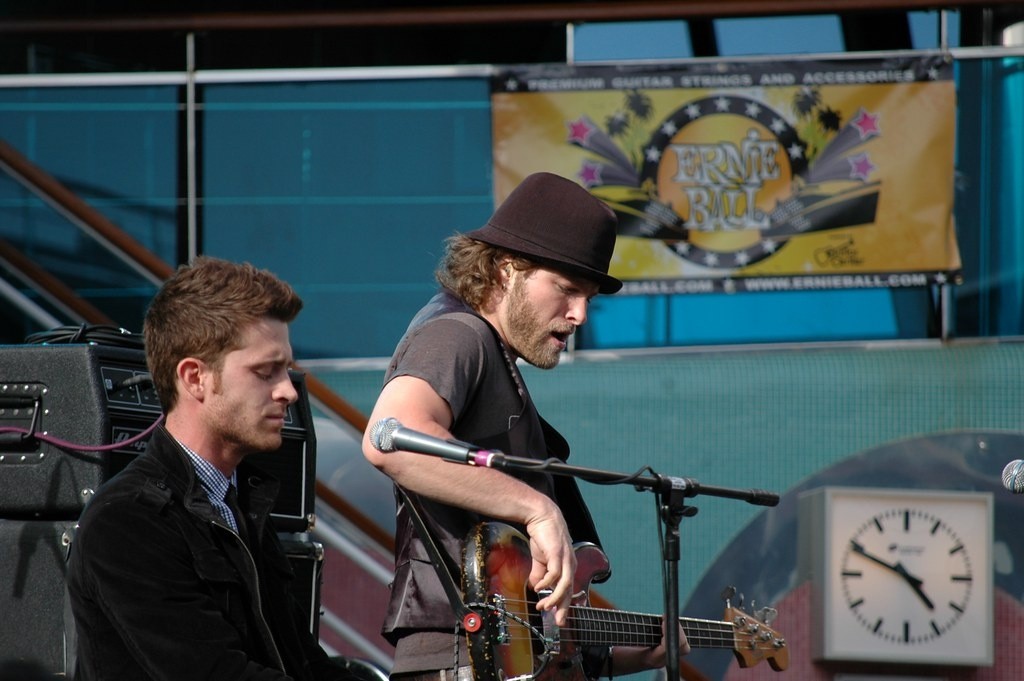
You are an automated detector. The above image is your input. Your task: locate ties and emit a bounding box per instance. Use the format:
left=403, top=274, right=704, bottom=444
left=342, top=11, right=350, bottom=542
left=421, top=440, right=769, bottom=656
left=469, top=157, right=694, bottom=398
left=224, top=482, right=252, bottom=558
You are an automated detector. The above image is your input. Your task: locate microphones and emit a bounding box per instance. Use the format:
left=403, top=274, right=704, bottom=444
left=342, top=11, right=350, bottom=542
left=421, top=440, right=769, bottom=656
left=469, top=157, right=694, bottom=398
left=1002, top=459, right=1024, bottom=492
left=370, top=417, right=510, bottom=468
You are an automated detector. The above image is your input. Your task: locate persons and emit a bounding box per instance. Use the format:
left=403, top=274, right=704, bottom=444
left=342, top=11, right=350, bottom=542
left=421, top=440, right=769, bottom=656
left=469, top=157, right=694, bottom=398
left=362, top=172, right=690, bottom=681
left=66, top=256, right=379, bottom=681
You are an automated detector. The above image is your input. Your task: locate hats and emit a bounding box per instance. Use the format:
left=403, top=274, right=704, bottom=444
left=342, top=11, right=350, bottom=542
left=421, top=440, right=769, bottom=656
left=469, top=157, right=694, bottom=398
left=464, top=171, right=623, bottom=295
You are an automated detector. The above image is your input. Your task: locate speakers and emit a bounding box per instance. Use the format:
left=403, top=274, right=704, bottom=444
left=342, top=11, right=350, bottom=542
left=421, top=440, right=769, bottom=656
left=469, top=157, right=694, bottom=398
left=0, top=518, right=325, bottom=681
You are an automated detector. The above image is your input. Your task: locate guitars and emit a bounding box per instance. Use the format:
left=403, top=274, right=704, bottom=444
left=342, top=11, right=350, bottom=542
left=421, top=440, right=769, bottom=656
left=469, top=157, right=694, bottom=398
left=460, top=520, right=791, bottom=680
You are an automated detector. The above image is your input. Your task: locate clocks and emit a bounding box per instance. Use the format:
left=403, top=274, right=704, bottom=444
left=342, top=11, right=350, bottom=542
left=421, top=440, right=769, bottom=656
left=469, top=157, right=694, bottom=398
left=810, top=486, right=995, bottom=666
